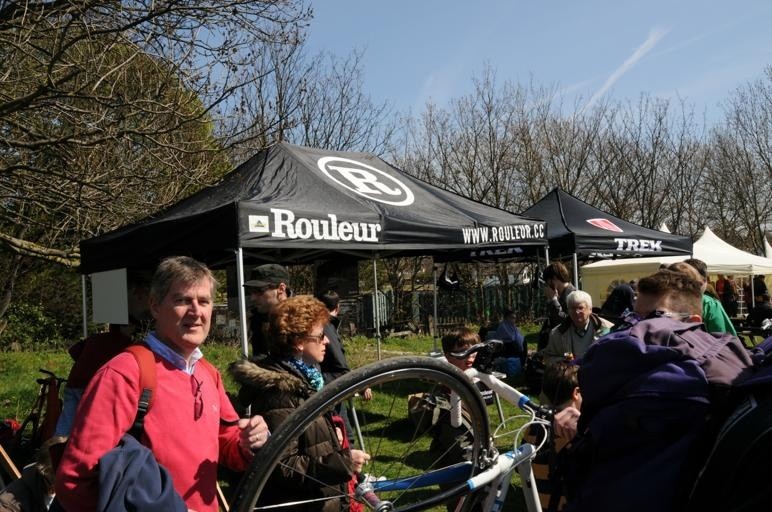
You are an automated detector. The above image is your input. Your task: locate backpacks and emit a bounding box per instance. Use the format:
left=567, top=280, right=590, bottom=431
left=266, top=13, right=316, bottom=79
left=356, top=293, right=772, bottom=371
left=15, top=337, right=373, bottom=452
left=564, top=311, right=772, bottom=512
left=51, top=332, right=218, bottom=469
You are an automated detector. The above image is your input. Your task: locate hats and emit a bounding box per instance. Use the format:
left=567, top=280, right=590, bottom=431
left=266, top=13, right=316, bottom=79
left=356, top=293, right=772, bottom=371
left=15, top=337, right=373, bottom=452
left=244, top=264, right=288, bottom=288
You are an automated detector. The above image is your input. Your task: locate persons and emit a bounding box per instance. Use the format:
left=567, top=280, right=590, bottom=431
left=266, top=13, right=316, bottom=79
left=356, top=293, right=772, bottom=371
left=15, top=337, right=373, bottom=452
left=433, top=328, right=498, bottom=511
left=318, top=289, right=388, bottom=487
left=230, top=293, right=371, bottom=510
left=0, top=435, right=74, bottom=511
left=237, top=264, right=375, bottom=448
left=473, top=258, right=772, bottom=510
left=55, top=253, right=272, bottom=512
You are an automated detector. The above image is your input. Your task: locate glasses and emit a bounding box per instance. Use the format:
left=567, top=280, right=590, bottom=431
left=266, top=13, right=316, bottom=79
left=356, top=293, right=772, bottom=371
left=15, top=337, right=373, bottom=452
left=244, top=286, right=270, bottom=300
left=191, top=375, right=205, bottom=420
left=295, top=329, right=326, bottom=346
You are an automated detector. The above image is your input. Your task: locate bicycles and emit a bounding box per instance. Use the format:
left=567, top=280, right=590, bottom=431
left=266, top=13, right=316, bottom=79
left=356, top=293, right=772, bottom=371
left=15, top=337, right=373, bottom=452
left=229, top=340, right=559, bottom=512
left=19, top=368, right=68, bottom=449
